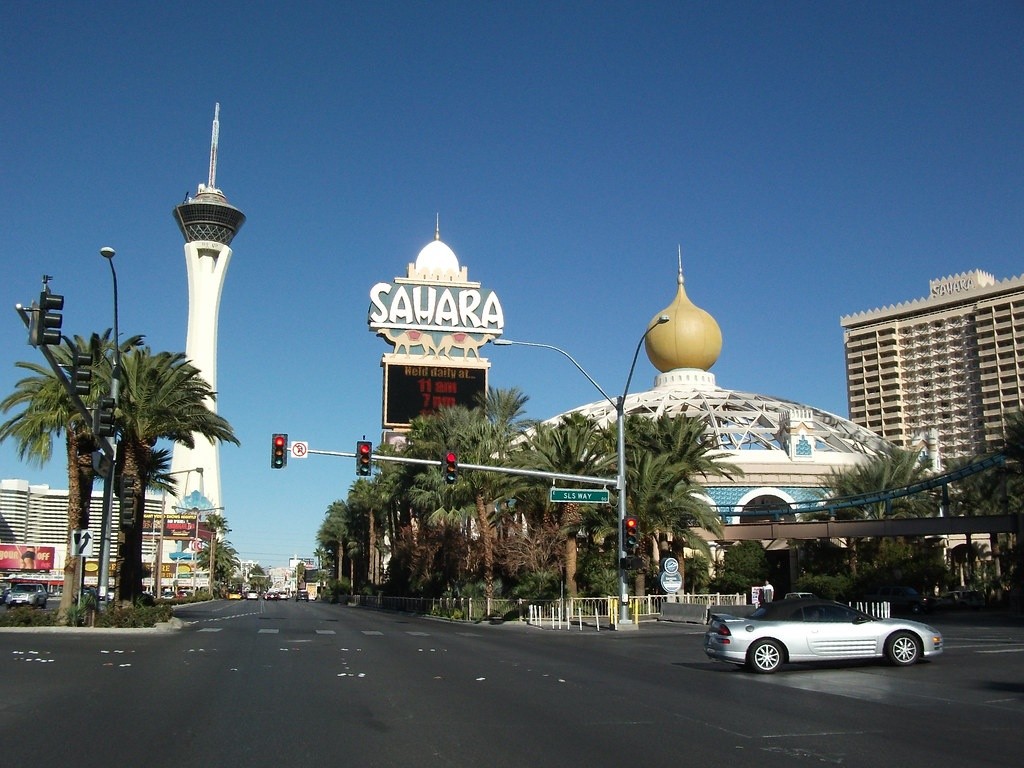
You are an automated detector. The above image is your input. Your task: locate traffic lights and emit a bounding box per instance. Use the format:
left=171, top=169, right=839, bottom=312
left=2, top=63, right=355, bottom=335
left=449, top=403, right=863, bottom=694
left=271, top=434, right=287, bottom=469
left=37, top=292, right=64, bottom=346
left=442, top=450, right=458, bottom=484
left=71, top=350, right=92, bottom=395
left=356, top=441, right=372, bottom=476
left=624, top=516, right=638, bottom=549
left=94, top=395, right=115, bottom=436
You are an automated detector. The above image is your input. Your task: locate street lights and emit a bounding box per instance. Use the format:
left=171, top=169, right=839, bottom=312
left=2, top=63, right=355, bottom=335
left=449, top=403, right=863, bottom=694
left=156, top=468, right=204, bottom=598
left=209, top=529, right=232, bottom=594
left=97, top=247, right=121, bottom=610
left=172, top=506, right=225, bottom=597
left=493, top=315, right=670, bottom=630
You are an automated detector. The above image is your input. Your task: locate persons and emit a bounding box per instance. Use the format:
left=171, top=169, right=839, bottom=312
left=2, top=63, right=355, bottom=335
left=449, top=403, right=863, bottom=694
left=21, top=546, right=36, bottom=568
left=808, top=606, right=820, bottom=619
left=763, top=580, right=774, bottom=602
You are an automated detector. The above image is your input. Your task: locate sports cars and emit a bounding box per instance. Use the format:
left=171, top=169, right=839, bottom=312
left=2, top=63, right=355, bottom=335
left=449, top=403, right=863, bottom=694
left=702, top=599, right=944, bottom=675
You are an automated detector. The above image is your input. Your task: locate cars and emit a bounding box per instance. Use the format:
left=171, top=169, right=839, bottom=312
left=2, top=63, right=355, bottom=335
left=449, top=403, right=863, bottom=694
left=164, top=592, right=176, bottom=599
left=246, top=590, right=259, bottom=600
left=226, top=591, right=241, bottom=600
left=5, top=584, right=48, bottom=609
left=263, top=592, right=289, bottom=600
left=947, top=591, right=981, bottom=611
left=296, top=590, right=310, bottom=602
left=876, top=587, right=941, bottom=616
left=83, top=587, right=114, bottom=600
left=784, top=593, right=818, bottom=599
